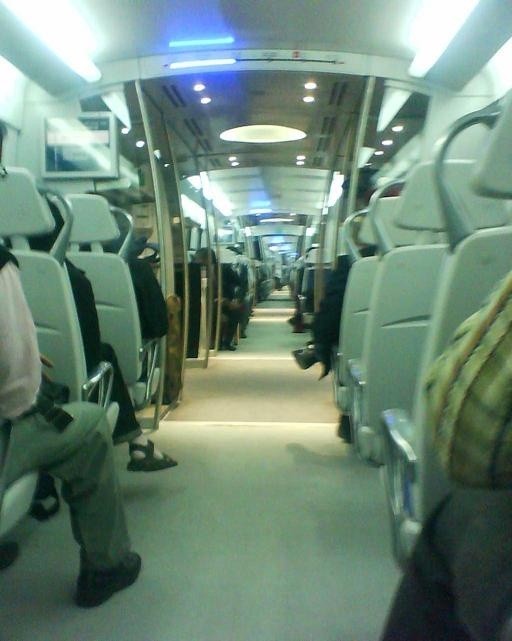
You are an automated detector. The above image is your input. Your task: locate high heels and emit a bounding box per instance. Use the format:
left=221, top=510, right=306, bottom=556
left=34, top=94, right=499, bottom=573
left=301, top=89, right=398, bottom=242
left=294, top=347, right=332, bottom=379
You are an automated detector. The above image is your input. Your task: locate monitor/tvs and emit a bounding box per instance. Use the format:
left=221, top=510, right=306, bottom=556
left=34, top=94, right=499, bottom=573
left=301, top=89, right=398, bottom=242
left=213, top=225, right=236, bottom=244
left=40, top=112, right=117, bottom=179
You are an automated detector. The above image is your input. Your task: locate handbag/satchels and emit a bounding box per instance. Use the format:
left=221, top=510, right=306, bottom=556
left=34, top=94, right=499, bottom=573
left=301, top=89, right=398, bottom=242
left=22, top=378, right=69, bottom=416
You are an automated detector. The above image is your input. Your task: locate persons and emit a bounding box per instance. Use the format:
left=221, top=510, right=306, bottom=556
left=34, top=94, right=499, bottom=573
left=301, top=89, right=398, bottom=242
left=378, top=271, right=510, bottom=640
left=291, top=248, right=381, bottom=382
left=191, top=246, right=317, bottom=352
left=0, top=123, right=142, bottom=607
left=125, top=258, right=173, bottom=407
left=64, top=257, right=179, bottom=472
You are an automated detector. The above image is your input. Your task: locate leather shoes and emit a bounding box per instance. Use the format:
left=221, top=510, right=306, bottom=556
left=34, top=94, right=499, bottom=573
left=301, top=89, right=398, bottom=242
left=77, top=552, right=140, bottom=608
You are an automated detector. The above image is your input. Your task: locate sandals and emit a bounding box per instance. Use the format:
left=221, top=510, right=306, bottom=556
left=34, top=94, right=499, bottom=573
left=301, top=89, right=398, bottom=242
left=127, top=440, right=178, bottom=470
